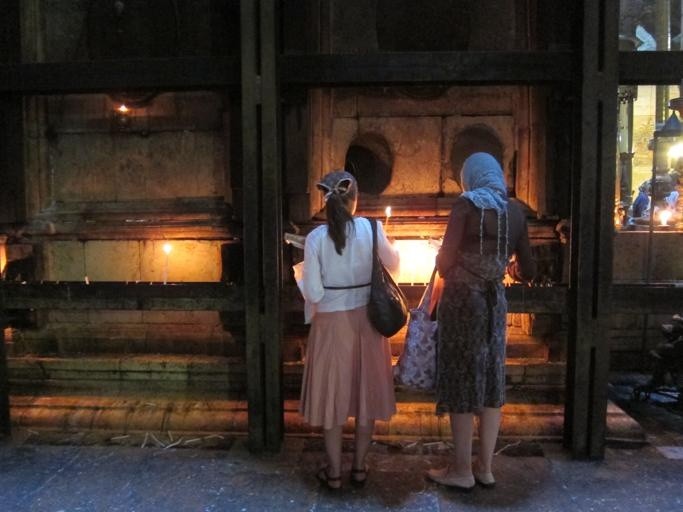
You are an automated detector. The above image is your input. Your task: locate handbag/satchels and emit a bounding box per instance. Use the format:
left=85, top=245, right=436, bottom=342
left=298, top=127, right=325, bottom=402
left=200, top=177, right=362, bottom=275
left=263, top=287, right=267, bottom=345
left=363, top=215, right=409, bottom=340
left=393, top=263, right=443, bottom=391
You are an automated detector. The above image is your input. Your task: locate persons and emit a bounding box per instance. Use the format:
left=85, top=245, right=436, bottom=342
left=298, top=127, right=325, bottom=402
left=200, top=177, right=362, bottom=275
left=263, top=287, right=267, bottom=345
left=297, top=170, right=401, bottom=492
left=425, top=151, right=538, bottom=492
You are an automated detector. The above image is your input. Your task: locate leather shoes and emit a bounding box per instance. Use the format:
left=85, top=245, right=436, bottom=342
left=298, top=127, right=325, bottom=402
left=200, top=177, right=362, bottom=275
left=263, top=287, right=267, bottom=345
left=427, top=464, right=476, bottom=493
left=472, top=462, right=497, bottom=488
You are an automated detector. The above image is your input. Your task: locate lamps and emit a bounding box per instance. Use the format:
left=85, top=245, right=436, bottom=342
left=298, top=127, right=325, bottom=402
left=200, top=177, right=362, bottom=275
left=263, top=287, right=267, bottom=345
left=635, top=108, right=681, bottom=372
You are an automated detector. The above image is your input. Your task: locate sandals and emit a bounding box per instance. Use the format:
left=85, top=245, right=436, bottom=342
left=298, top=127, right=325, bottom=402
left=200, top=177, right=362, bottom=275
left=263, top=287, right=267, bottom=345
left=350, top=461, right=370, bottom=487
left=314, top=463, right=344, bottom=493
